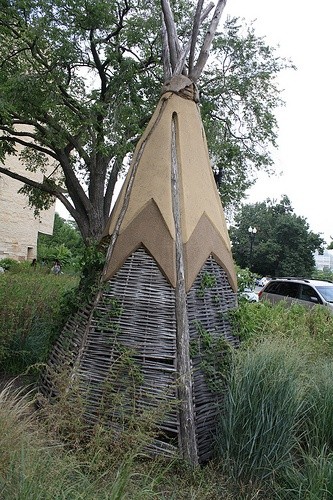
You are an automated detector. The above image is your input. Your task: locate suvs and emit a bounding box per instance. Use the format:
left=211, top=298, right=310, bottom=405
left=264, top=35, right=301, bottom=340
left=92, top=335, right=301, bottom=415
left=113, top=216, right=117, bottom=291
left=258, top=278, right=333, bottom=316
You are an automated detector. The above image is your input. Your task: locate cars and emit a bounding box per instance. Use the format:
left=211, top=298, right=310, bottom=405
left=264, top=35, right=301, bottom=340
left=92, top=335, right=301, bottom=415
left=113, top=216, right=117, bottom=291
left=242, top=281, right=265, bottom=303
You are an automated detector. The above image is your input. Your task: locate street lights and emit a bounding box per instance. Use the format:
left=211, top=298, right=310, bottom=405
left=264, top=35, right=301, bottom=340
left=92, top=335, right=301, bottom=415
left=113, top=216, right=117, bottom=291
left=247, top=225, right=257, bottom=277
